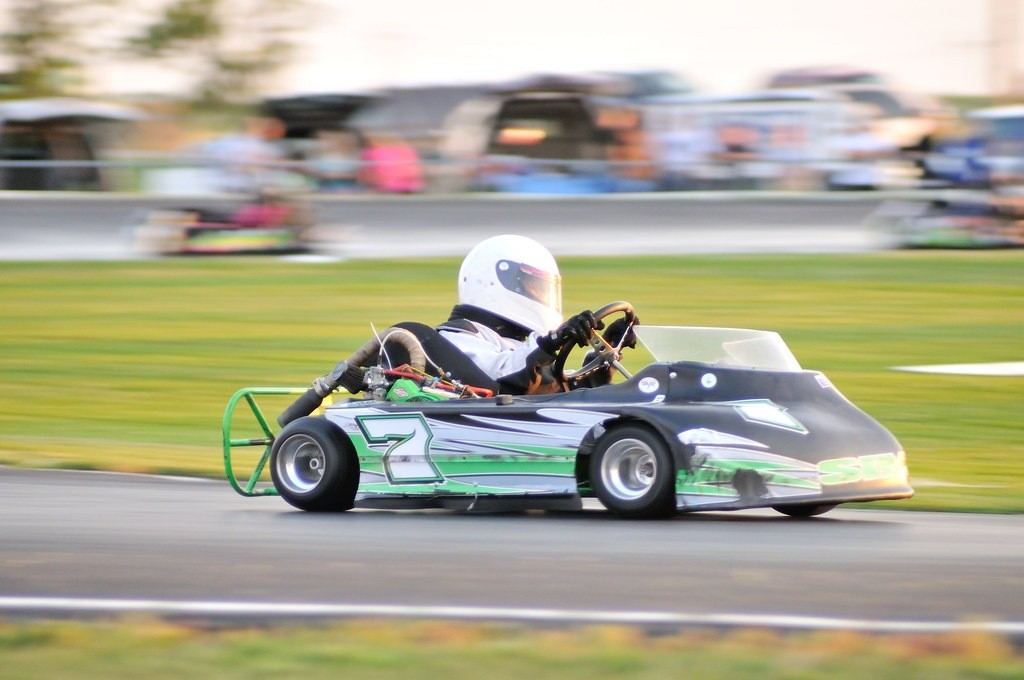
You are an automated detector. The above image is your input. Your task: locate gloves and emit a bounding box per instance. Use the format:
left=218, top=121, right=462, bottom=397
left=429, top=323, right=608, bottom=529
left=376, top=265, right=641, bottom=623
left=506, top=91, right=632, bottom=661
left=595, top=315, right=640, bottom=351
left=537, top=310, right=597, bottom=357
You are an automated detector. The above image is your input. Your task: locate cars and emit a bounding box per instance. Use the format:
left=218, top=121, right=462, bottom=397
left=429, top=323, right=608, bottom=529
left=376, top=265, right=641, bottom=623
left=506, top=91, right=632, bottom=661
left=1, top=68, right=1023, bottom=193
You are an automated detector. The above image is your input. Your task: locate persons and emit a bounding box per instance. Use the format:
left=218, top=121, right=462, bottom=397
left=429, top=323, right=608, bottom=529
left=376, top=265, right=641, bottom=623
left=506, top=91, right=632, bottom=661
left=148, top=116, right=421, bottom=258
left=435, top=235, right=642, bottom=397
left=593, top=105, right=1024, bottom=216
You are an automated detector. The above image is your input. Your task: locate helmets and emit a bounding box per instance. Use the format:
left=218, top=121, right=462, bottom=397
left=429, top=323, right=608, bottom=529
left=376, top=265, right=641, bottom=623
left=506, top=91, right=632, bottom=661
left=458, top=235, right=563, bottom=338
